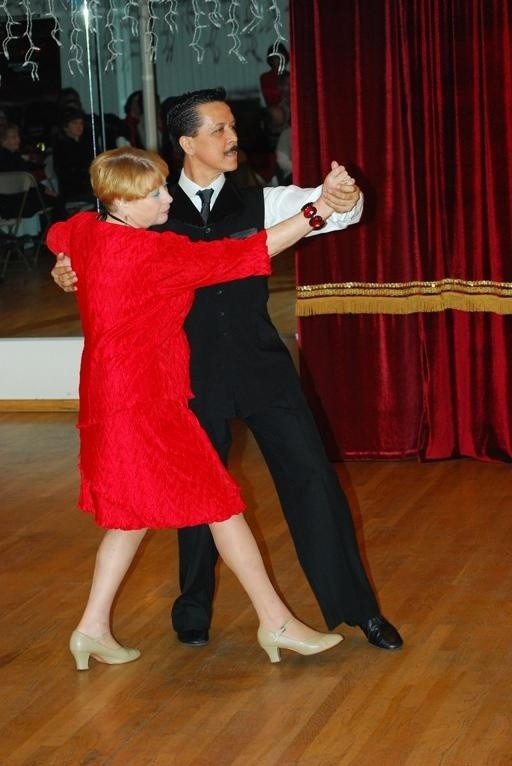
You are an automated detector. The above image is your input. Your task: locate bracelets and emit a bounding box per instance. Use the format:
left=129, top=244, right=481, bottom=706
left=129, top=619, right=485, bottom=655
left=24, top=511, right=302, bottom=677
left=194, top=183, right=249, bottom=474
left=301, top=202, right=326, bottom=231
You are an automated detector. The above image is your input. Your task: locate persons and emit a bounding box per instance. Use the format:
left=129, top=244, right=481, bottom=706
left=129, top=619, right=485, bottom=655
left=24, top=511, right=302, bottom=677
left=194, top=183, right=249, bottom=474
left=46, top=146, right=356, bottom=673
left=51, top=90, right=403, bottom=651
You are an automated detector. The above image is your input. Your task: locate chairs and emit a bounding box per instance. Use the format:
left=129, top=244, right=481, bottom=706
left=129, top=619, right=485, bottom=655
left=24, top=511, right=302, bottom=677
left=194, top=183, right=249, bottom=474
left=0, top=171, right=58, bottom=278
left=40, top=146, right=95, bottom=214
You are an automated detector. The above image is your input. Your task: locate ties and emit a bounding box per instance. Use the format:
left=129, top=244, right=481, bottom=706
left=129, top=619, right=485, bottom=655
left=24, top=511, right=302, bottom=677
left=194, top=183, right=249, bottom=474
left=196, top=189, right=214, bottom=226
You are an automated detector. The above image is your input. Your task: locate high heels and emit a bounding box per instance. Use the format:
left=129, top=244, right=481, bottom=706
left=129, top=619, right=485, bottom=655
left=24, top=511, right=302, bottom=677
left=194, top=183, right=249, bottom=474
left=69, top=630, right=142, bottom=671
left=256, top=616, right=344, bottom=663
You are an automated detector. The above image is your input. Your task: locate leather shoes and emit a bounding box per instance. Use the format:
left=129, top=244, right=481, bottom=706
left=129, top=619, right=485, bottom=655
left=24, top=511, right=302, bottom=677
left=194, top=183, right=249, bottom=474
left=177, top=629, right=209, bottom=648
left=345, top=609, right=405, bottom=653
